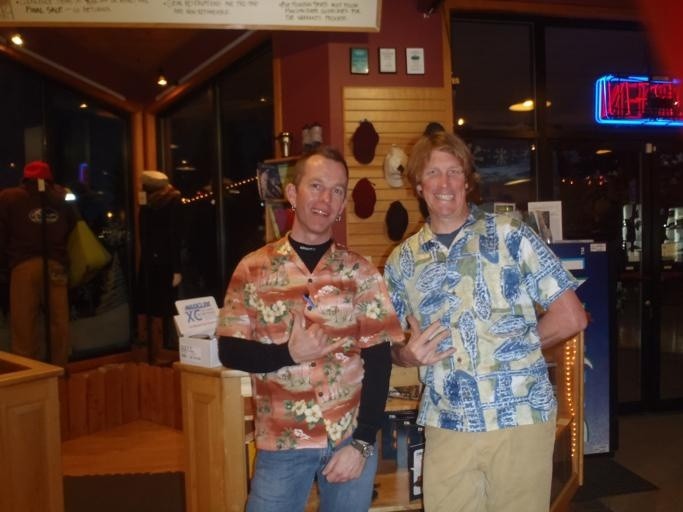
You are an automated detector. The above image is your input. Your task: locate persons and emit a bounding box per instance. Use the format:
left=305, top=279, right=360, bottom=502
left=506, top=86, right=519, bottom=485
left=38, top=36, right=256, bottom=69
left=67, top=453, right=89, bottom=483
left=136, top=170, right=183, bottom=366
left=213, top=144, right=406, bottom=512
left=380, top=129, right=590, bottom=511
left=0, top=159, right=74, bottom=379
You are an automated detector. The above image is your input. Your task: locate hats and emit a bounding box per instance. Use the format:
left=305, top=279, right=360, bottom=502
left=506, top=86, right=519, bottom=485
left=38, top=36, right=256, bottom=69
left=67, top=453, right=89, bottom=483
left=24, top=161, right=52, bottom=181
left=384, top=146, right=408, bottom=188
left=385, top=201, right=408, bottom=241
left=353, top=178, right=376, bottom=218
left=419, top=199, right=429, bottom=217
left=426, top=123, right=444, bottom=134
left=352, top=121, right=378, bottom=163
left=142, top=171, right=168, bottom=192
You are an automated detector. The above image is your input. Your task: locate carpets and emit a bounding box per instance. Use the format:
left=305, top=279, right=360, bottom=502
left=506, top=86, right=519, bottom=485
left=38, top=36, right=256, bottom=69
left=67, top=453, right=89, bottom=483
left=552, top=457, right=658, bottom=501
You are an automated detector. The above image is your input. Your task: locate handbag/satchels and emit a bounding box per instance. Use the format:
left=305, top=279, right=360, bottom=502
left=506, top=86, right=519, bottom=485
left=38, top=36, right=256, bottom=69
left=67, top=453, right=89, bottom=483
left=67, top=220, right=104, bottom=287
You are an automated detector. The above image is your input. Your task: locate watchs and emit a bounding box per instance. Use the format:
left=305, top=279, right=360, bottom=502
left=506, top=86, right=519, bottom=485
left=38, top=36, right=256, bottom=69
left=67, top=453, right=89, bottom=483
left=349, top=439, right=374, bottom=459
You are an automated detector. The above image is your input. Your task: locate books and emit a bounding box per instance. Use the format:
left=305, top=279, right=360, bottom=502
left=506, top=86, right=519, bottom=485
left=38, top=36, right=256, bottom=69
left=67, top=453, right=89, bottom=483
left=526, top=200, right=562, bottom=241
left=173, top=295, right=220, bottom=338
